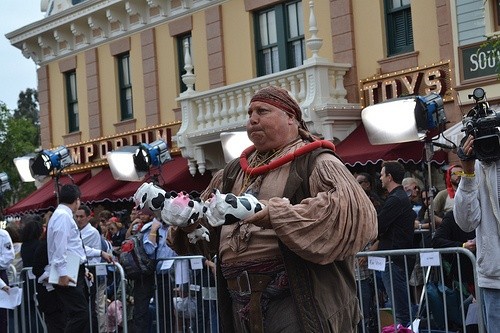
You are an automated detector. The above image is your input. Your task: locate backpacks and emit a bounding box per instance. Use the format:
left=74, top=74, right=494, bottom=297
left=121, top=231, right=153, bottom=277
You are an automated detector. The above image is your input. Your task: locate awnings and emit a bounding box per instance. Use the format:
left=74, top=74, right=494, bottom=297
left=112, top=156, right=214, bottom=203
left=79, top=166, right=129, bottom=204
left=335, top=121, right=446, bottom=168
left=5, top=172, right=92, bottom=212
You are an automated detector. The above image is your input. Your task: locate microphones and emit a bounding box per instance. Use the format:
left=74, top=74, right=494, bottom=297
left=467, top=122, right=473, bottom=130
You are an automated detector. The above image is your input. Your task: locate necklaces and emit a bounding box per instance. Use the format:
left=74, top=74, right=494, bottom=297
left=237, top=132, right=303, bottom=197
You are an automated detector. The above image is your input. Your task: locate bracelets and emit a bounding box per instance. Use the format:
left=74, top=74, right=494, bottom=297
left=463, top=242, right=466, bottom=249
left=100, top=249, right=102, bottom=256
left=462, top=173, right=476, bottom=178
left=149, top=232, right=157, bottom=237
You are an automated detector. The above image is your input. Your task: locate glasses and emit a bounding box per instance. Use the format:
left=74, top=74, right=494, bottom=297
left=423, top=197, right=430, bottom=202
left=101, top=220, right=107, bottom=223
left=358, top=180, right=365, bottom=184
left=108, top=227, right=116, bottom=231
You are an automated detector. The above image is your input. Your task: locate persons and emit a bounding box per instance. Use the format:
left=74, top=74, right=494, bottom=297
left=1, top=185, right=217, bottom=332
left=454, top=104, right=500, bottom=333
left=166, top=86, right=378, bottom=333
left=350, top=159, right=479, bottom=333
